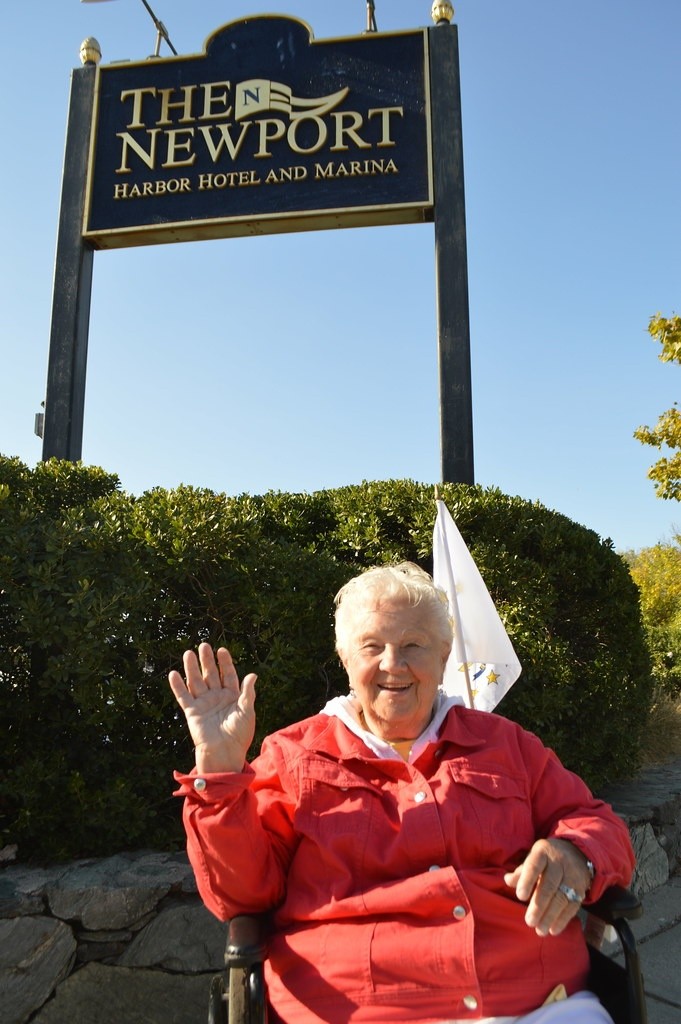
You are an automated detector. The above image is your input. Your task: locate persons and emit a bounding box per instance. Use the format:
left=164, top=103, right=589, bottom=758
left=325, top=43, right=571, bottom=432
left=168, top=563, right=637, bottom=1024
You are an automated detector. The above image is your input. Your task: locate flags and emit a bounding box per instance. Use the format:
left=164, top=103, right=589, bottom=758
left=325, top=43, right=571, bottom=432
left=433, top=502, right=521, bottom=713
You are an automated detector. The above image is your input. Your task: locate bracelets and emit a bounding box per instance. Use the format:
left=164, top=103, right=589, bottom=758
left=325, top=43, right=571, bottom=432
left=586, top=860, right=594, bottom=890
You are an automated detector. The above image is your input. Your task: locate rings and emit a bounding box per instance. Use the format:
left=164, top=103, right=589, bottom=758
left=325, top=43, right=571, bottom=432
left=560, top=883, right=576, bottom=903
left=576, top=895, right=582, bottom=903
